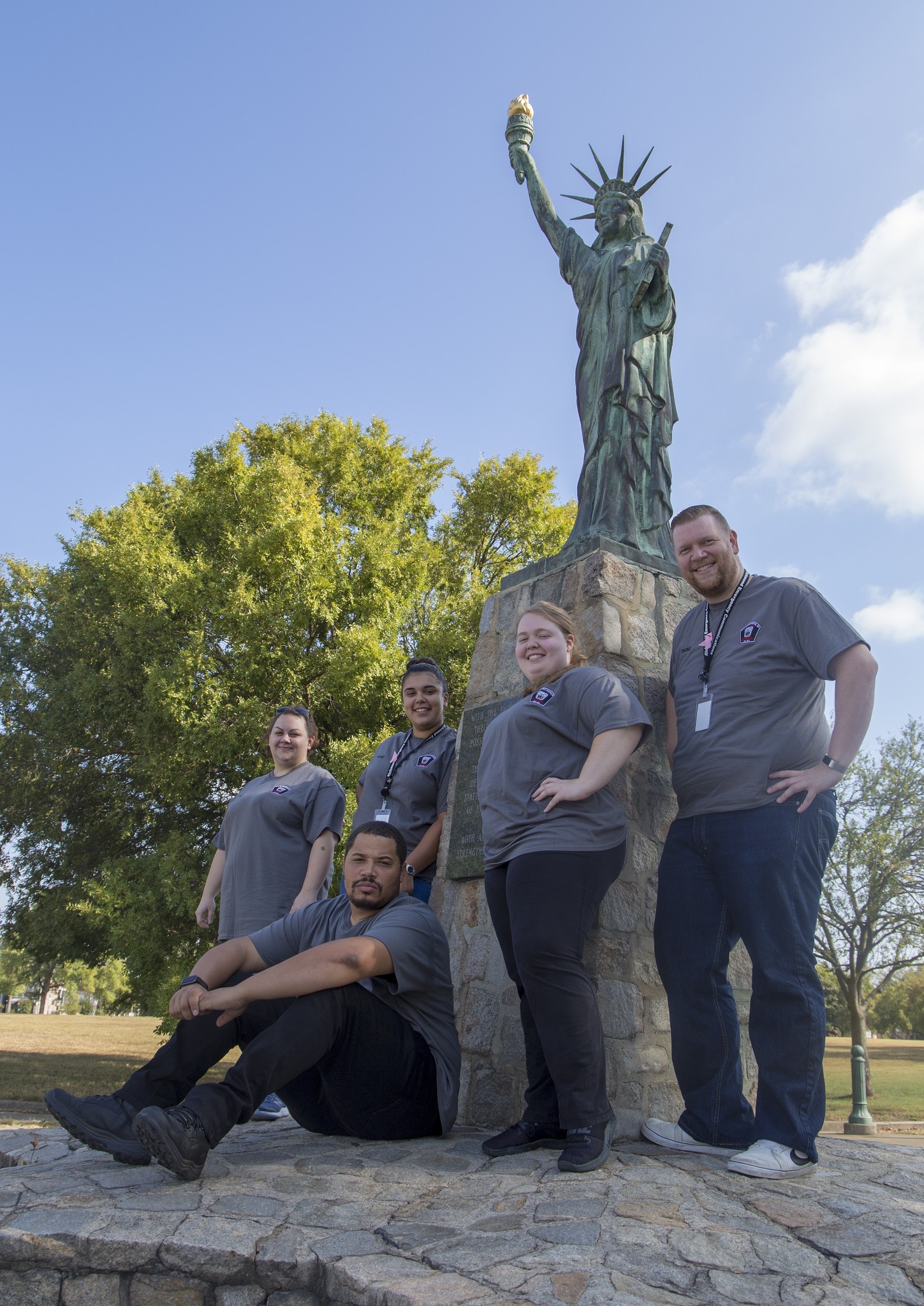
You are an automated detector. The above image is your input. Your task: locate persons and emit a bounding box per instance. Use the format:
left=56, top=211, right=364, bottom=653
left=474, top=600, right=652, bottom=1171
left=190, top=706, right=346, bottom=1122
left=46, top=820, right=462, bottom=1181
left=350, top=652, right=463, bottom=901
left=506, top=108, right=684, bottom=563
left=643, top=504, right=879, bottom=1180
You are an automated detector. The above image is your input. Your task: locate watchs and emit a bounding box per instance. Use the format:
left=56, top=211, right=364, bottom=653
left=179, top=975, right=209, bottom=992
left=403, top=862, right=416, bottom=876
left=822, top=753, right=849, bottom=775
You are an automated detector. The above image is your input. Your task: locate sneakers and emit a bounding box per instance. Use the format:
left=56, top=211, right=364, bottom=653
left=250, top=1092, right=290, bottom=1121
left=640, top=1117, right=747, bottom=1158
left=728, top=1138, right=818, bottom=1180
left=558, top=1111, right=617, bottom=1172
left=481, top=1118, right=566, bottom=1156
left=134, top=1104, right=206, bottom=1182
left=44, top=1089, right=152, bottom=1166
